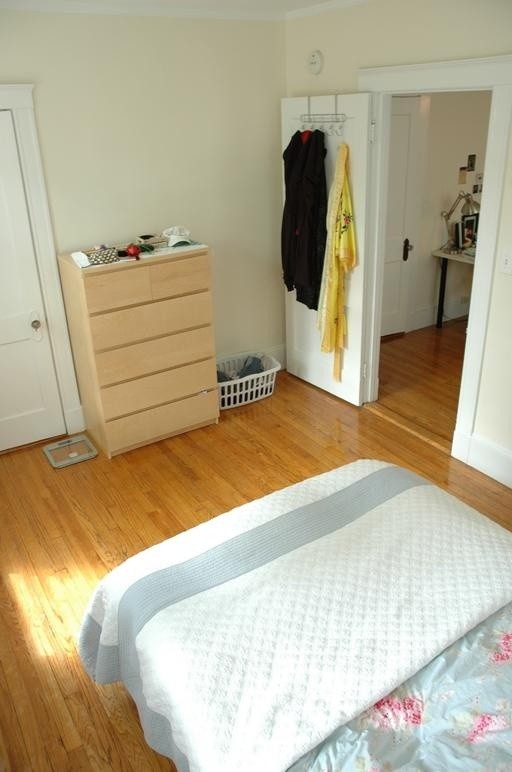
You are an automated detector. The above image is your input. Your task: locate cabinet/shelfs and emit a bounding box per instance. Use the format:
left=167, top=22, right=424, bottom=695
left=55, top=233, right=221, bottom=460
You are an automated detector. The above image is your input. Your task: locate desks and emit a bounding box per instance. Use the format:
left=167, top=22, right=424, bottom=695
left=432, top=245, right=474, bottom=330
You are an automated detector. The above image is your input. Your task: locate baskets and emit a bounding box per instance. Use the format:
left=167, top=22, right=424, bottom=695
left=216, top=351, right=281, bottom=410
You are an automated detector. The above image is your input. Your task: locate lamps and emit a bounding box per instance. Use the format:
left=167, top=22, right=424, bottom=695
left=441, top=191, right=481, bottom=255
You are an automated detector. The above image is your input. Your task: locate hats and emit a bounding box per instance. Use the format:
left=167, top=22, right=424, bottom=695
left=160, top=226, right=192, bottom=247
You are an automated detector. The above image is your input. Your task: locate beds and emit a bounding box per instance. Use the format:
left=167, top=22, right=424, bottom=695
left=114, top=457, right=512, bottom=771
